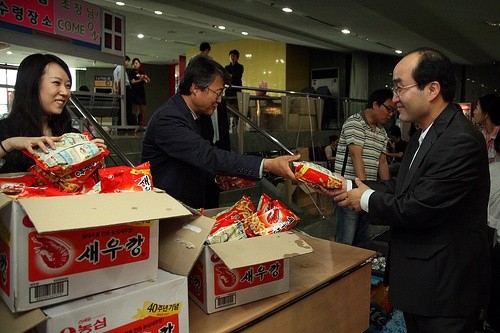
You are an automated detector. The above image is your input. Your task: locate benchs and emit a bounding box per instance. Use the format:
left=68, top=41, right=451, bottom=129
left=66, top=97, right=119, bottom=136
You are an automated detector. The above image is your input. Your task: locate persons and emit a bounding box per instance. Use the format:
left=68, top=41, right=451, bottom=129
left=313, top=48, right=489, bottom=333
left=112, top=56, right=150, bottom=135
left=141, top=55, right=301, bottom=212
left=223, top=49, right=245, bottom=131
left=0, top=53, right=82, bottom=173
left=322, top=86, right=406, bottom=248
left=78, top=86, right=91, bottom=101
left=189, top=42, right=215, bottom=62
left=472, top=95, right=500, bottom=254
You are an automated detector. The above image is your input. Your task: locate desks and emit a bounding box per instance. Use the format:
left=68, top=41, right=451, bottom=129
left=188, top=228, right=376, bottom=333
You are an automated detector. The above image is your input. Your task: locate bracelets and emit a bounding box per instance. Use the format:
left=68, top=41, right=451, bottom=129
left=0, top=140, right=8, bottom=154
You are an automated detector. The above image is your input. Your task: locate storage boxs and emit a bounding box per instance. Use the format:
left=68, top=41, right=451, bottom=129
left=0, top=172, right=314, bottom=333
left=250, top=96, right=318, bottom=132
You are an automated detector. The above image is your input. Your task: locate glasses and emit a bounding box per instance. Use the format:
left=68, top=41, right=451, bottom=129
left=382, top=103, right=396, bottom=113
left=391, top=82, right=419, bottom=96
left=205, top=86, right=224, bottom=97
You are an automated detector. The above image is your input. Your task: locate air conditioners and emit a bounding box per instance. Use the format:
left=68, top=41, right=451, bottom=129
left=310, top=66, right=346, bottom=118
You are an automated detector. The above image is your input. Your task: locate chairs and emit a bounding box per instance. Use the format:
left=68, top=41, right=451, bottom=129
left=301, top=86, right=334, bottom=130
